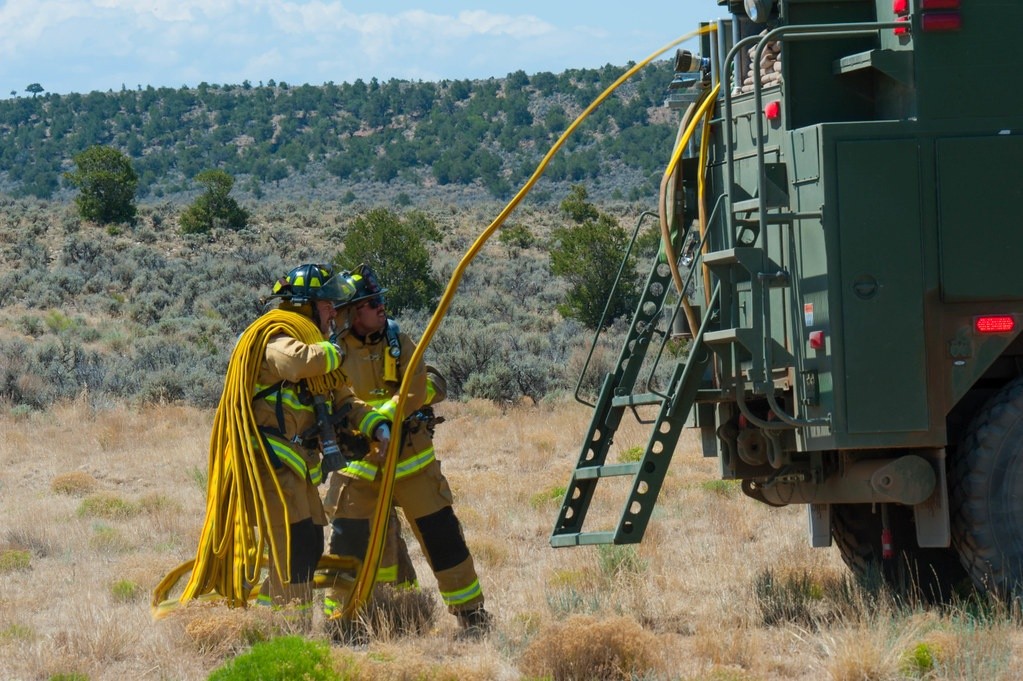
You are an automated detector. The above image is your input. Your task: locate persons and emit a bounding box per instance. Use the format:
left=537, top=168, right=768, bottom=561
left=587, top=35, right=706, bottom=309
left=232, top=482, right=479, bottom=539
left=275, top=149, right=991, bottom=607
left=322, top=265, right=488, bottom=631
left=244, top=264, right=394, bottom=627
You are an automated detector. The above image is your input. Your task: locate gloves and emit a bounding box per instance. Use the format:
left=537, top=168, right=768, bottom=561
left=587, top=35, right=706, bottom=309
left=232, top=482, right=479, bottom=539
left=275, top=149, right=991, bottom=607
left=332, top=344, right=346, bottom=369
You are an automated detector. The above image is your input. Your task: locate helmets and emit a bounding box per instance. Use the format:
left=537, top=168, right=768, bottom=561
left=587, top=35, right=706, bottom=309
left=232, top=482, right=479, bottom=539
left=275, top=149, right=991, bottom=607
left=331, top=263, right=390, bottom=308
left=267, top=264, right=342, bottom=302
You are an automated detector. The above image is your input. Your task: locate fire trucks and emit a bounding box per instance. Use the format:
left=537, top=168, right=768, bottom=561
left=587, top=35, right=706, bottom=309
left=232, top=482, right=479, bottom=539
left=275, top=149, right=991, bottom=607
left=548, top=0, right=1023, bottom=614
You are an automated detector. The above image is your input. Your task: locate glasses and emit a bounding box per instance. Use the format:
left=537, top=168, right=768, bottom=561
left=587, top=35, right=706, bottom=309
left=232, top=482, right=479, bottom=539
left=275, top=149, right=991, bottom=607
left=357, top=295, right=387, bottom=310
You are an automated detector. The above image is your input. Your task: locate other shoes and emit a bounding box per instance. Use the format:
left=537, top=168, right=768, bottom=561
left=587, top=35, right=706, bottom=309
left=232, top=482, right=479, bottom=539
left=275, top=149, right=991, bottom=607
left=458, top=612, right=491, bottom=628
